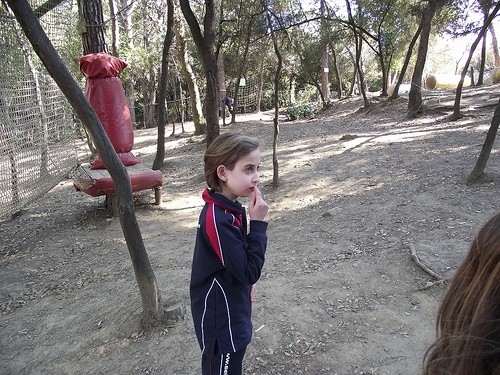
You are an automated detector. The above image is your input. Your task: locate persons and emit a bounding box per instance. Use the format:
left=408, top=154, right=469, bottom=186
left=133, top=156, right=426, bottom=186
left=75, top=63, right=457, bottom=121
left=421, top=213, right=500, bottom=375
left=223, top=96, right=234, bottom=114
left=189, top=134, right=268, bottom=375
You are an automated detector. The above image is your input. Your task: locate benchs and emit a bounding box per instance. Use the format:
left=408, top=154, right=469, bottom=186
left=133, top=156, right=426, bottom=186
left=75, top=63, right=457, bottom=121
left=79, top=162, right=163, bottom=217
